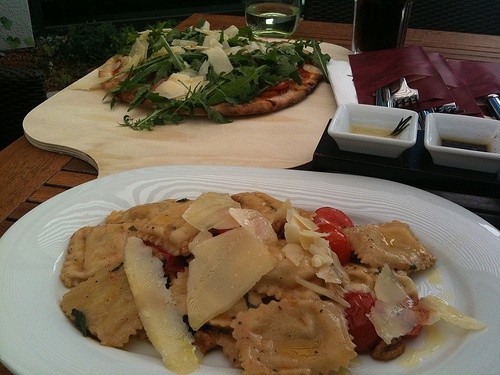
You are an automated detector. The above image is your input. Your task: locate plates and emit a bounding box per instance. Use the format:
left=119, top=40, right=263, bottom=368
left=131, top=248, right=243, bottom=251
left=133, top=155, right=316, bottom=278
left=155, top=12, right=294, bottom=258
left=0, top=166, right=500, bottom=375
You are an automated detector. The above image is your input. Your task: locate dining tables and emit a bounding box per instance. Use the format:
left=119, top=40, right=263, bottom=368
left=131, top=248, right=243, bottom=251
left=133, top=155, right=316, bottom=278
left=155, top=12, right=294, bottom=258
left=0, top=13, right=500, bottom=242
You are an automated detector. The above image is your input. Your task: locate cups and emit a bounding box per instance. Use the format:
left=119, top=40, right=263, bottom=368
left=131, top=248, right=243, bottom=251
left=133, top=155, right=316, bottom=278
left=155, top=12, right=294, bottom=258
left=245, top=0, right=304, bottom=39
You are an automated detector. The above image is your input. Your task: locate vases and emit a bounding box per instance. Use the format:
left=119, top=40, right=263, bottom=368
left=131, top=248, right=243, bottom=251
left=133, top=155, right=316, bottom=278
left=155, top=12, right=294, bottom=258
left=7, top=74, right=32, bottom=95
left=46, top=91, right=58, bottom=97
left=28, top=78, right=46, bottom=94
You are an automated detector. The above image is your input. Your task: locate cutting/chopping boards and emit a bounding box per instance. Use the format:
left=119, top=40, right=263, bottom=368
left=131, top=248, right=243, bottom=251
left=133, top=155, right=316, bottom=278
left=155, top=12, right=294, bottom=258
left=22, top=29, right=352, bottom=182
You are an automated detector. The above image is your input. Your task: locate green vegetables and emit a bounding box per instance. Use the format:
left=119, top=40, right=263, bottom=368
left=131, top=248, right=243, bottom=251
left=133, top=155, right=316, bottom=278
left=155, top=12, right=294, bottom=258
left=100, top=16, right=331, bottom=131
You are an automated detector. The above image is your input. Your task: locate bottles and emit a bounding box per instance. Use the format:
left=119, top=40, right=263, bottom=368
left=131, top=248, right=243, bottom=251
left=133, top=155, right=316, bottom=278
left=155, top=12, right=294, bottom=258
left=352, top=0, right=413, bottom=53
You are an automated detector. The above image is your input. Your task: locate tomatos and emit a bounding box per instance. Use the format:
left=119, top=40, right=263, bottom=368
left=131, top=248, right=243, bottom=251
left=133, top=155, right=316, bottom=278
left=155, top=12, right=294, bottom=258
left=338, top=291, right=379, bottom=352
left=312, top=207, right=357, bottom=264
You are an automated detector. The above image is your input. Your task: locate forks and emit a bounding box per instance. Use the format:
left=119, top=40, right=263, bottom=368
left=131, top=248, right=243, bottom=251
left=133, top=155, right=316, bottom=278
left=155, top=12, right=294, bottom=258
left=387, top=77, right=419, bottom=111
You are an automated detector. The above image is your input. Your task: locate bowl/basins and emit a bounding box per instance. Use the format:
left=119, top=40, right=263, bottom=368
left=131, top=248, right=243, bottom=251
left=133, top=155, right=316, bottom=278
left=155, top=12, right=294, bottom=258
left=424, top=113, right=500, bottom=174
left=327, top=103, right=419, bottom=159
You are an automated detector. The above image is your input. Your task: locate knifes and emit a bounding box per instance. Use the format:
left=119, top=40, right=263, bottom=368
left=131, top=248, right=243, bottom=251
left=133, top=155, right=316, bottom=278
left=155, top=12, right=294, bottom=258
left=376, top=87, right=390, bottom=107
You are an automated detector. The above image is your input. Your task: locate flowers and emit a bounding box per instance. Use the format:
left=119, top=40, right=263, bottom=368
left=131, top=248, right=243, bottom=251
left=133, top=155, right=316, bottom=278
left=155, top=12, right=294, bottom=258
left=0, top=34, right=73, bottom=98
